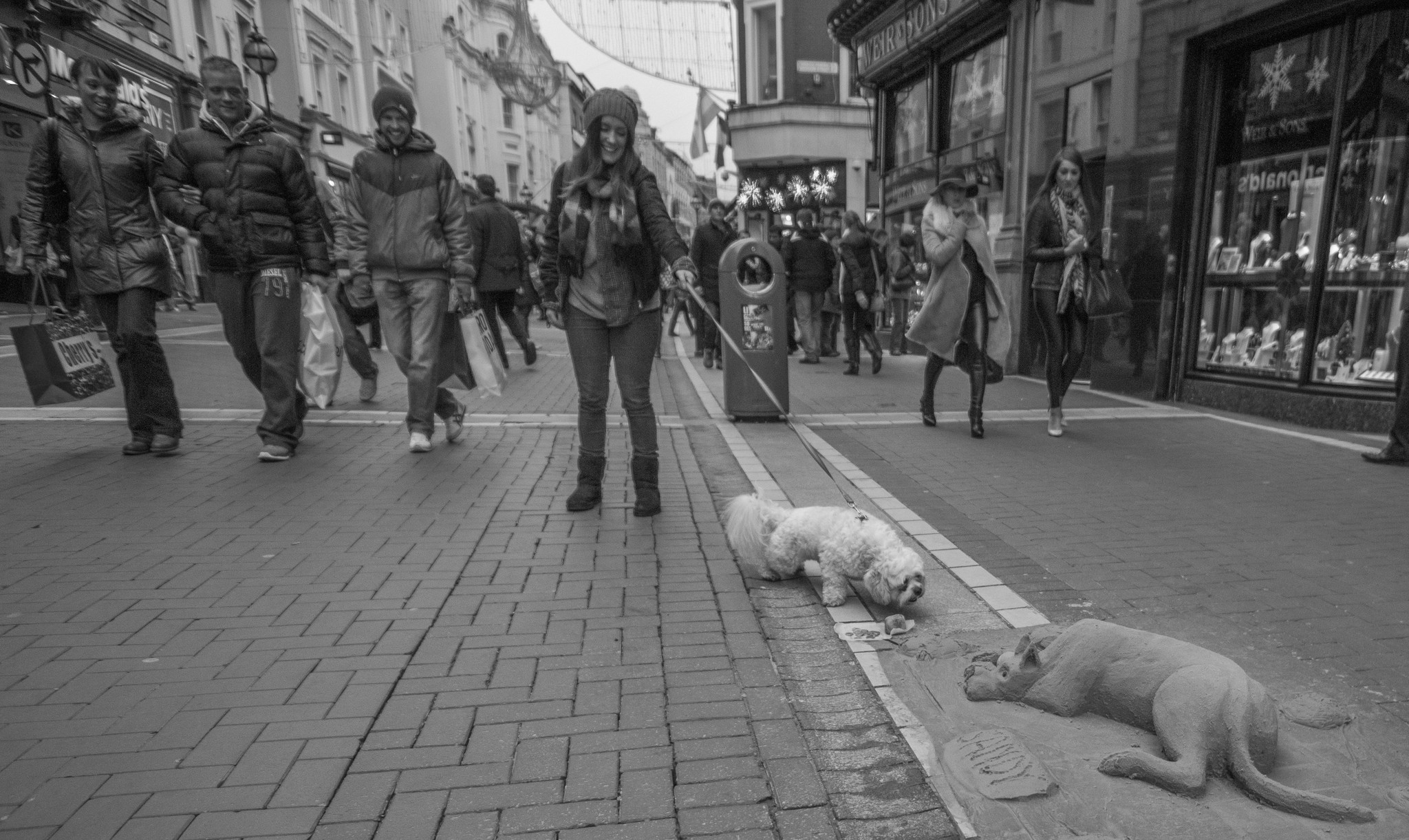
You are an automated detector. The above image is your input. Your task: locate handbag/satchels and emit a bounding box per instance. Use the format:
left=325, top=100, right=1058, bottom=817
left=5, top=245, right=24, bottom=274
left=300, top=281, right=344, bottom=408
left=870, top=296, right=884, bottom=312
left=1086, top=259, right=1133, bottom=321
left=459, top=305, right=507, bottom=398
left=10, top=274, right=115, bottom=406
left=170, top=270, right=186, bottom=292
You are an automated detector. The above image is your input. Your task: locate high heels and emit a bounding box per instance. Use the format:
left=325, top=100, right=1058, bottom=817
left=1047, top=395, right=1068, bottom=427
left=1048, top=427, right=1062, bottom=437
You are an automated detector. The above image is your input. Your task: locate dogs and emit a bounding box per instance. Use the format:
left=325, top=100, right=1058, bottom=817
left=716, top=491, right=926, bottom=608
left=959, top=618, right=1375, bottom=821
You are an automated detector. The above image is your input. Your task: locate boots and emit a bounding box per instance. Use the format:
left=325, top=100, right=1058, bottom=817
left=968, top=371, right=985, bottom=436
left=920, top=362, right=944, bottom=426
left=629, top=457, right=661, bottom=516
left=566, top=455, right=605, bottom=510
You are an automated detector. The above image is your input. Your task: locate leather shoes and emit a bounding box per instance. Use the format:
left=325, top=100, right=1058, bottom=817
left=1361, top=448, right=1409, bottom=466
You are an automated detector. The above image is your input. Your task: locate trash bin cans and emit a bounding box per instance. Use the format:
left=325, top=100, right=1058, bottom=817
left=718, top=237, right=789, bottom=420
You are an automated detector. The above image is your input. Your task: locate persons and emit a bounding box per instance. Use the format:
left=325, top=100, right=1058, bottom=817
left=10, top=203, right=83, bottom=316
left=346, top=85, right=473, bottom=452
left=154, top=57, right=329, bottom=460
left=661, top=199, right=756, bottom=368
left=158, top=220, right=195, bottom=311
left=769, top=208, right=916, bottom=376
left=1026, top=147, right=1103, bottom=435
left=905, top=164, right=1011, bottom=437
left=20, top=56, right=184, bottom=455
left=469, top=174, right=560, bottom=368
left=307, top=173, right=382, bottom=408
left=538, top=88, right=697, bottom=515
left=1360, top=270, right=1409, bottom=463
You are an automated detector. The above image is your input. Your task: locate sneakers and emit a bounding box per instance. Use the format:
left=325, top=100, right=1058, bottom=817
left=122, top=340, right=537, bottom=461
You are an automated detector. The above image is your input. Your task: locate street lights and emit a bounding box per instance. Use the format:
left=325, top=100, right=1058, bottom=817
left=518, top=182, right=539, bottom=262
left=243, top=17, right=278, bottom=120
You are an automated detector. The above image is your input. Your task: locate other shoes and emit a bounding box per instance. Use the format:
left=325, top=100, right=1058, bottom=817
left=891, top=273, right=915, bottom=292
left=155, top=303, right=197, bottom=313
left=787, top=342, right=907, bottom=376
left=668, top=331, right=679, bottom=337
left=703, top=348, right=713, bottom=368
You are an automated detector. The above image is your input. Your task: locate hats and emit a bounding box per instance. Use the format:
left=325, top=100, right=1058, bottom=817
left=708, top=198, right=726, bottom=214
left=929, top=165, right=978, bottom=197
left=372, top=87, right=417, bottom=125
left=583, top=89, right=638, bottom=143
